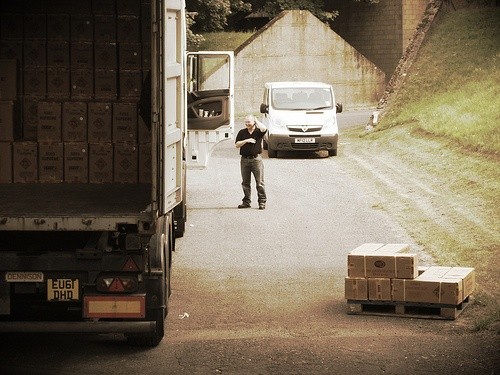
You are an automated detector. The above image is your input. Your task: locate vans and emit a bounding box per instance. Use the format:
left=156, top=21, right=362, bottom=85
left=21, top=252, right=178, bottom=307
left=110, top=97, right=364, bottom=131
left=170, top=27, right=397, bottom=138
left=259, top=82, right=342, bottom=159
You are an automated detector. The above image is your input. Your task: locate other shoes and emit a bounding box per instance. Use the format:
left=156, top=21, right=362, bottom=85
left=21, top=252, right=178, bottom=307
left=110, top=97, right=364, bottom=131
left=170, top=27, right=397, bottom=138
left=238, top=204, right=251, bottom=209
left=259, top=205, right=265, bottom=209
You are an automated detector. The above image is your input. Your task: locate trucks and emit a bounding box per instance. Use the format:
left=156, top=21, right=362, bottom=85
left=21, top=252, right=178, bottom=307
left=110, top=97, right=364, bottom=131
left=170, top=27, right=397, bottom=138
left=1, top=0, right=235, bottom=348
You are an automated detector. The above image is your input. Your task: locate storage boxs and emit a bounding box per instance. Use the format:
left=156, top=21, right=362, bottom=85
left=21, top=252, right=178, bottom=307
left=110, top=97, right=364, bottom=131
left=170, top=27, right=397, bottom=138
left=0, top=0, right=150, bottom=184
left=345, top=242, right=476, bottom=305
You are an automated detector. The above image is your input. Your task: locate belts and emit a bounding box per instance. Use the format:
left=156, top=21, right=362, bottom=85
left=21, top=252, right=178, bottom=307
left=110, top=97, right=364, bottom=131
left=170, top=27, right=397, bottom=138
left=242, top=154, right=258, bottom=159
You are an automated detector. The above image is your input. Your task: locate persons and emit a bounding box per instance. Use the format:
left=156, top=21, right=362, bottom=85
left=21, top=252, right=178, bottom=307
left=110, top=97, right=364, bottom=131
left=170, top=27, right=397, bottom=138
left=235, top=114, right=268, bottom=209
